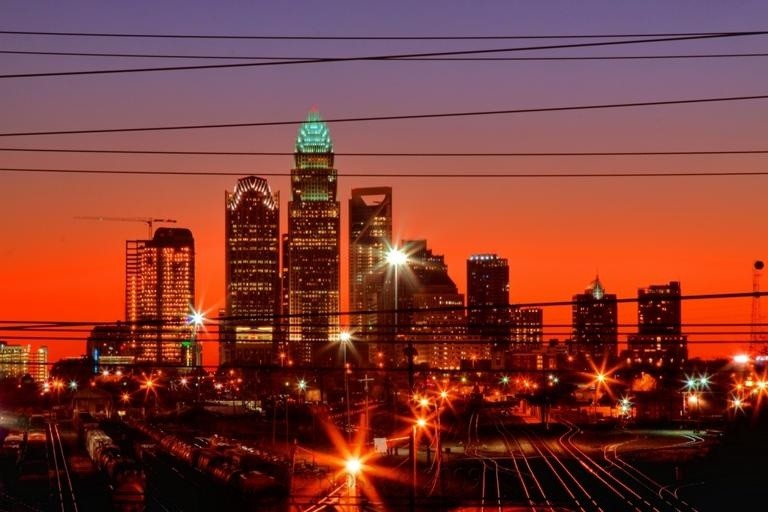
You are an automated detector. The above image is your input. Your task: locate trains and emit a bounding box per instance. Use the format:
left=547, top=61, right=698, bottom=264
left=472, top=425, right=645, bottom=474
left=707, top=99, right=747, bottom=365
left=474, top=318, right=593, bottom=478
left=0, top=406, right=290, bottom=512
